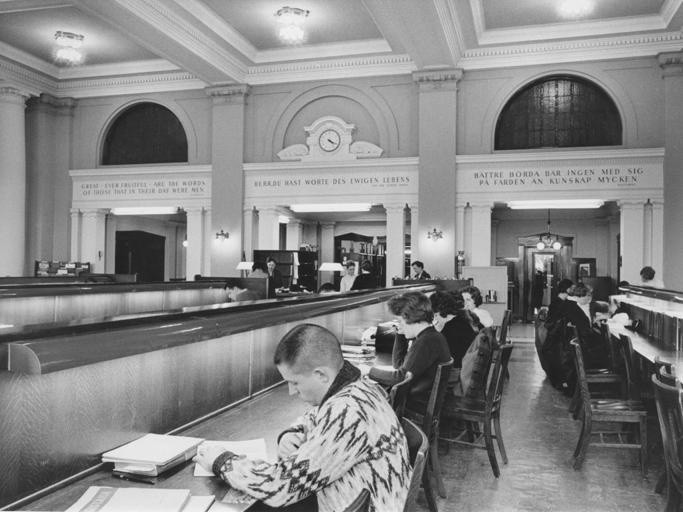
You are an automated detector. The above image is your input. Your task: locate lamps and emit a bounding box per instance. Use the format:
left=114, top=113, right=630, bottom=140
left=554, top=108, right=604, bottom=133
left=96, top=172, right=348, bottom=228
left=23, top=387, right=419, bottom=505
left=534, top=208, right=562, bottom=251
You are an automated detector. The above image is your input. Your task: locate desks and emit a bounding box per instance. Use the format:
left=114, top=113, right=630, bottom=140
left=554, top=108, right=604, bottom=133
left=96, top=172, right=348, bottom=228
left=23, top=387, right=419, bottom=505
left=0, top=380, right=319, bottom=512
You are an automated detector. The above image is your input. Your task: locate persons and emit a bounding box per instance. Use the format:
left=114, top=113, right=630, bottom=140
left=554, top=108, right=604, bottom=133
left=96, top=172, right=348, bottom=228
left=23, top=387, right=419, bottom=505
left=530, top=268, right=547, bottom=318
left=247, top=259, right=378, bottom=302
left=410, top=260, right=433, bottom=281
left=192, top=322, right=413, bottom=512
left=342, top=291, right=450, bottom=447
left=538, top=276, right=614, bottom=385
left=428, top=285, right=496, bottom=411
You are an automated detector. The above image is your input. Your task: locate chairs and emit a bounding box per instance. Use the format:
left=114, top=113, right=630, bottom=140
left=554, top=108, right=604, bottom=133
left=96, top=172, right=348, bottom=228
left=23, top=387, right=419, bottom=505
left=570, top=337, right=648, bottom=480
left=535, top=304, right=639, bottom=421
left=444, top=309, right=514, bottom=455
left=407, top=356, right=448, bottom=512
left=650, top=354, right=682, bottom=512
left=345, top=415, right=430, bottom=512
left=444, top=343, right=514, bottom=479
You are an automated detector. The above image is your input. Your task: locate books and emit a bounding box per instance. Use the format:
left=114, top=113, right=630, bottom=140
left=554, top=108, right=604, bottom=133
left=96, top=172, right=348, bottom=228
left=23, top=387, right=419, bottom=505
left=64, top=433, right=216, bottom=512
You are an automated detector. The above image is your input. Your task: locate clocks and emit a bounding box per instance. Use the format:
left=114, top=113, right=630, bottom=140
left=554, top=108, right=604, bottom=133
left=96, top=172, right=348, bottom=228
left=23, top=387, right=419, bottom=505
left=316, top=128, right=342, bottom=154
